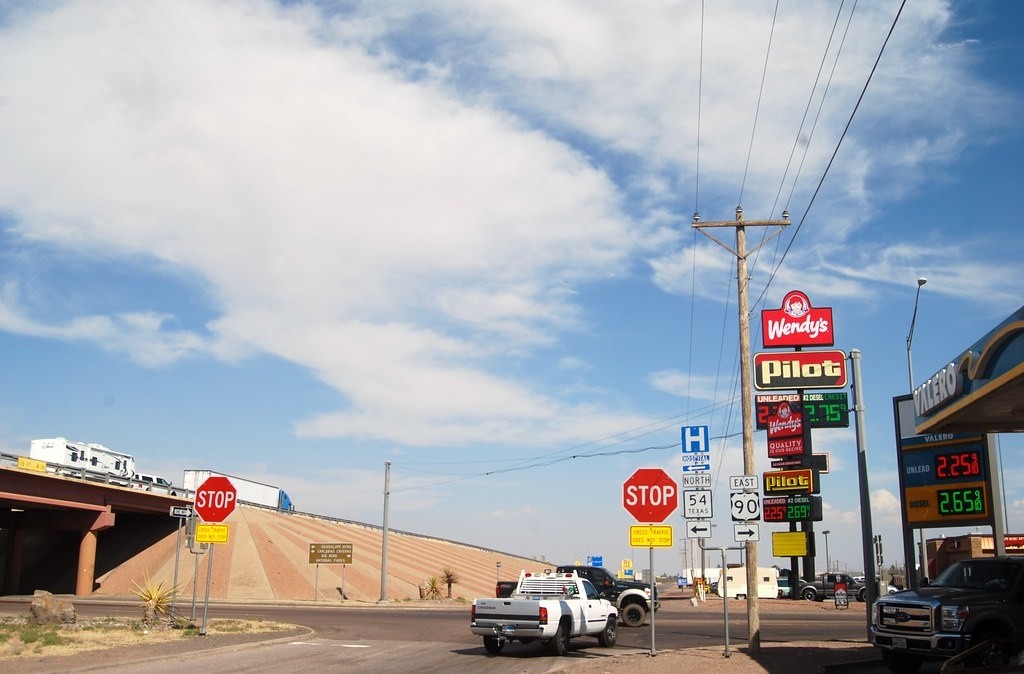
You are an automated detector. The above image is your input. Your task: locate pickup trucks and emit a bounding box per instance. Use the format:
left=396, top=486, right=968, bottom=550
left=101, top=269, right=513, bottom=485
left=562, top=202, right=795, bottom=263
left=496, top=565, right=662, bottom=628
left=797, top=572, right=867, bottom=602
left=470, top=569, right=620, bottom=656
left=869, top=555, right=1024, bottom=674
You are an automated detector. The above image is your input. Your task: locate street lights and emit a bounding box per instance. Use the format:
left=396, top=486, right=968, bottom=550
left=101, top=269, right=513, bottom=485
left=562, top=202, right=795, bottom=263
left=822, top=530, right=831, bottom=573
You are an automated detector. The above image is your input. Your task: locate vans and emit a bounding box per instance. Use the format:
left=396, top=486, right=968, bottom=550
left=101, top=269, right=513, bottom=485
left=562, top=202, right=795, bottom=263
left=128, top=473, right=178, bottom=496
left=852, top=577, right=865, bottom=583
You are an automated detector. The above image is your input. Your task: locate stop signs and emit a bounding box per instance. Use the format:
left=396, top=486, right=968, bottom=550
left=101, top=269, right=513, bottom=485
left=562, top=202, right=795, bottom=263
left=622, top=468, right=678, bottom=523
left=194, top=476, right=238, bottom=523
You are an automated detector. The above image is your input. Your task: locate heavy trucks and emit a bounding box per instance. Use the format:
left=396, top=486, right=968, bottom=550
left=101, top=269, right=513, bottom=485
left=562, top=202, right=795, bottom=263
left=182, top=469, right=295, bottom=515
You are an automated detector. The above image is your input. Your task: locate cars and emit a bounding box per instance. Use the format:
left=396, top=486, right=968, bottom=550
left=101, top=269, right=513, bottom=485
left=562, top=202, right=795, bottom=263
left=887, top=585, right=899, bottom=594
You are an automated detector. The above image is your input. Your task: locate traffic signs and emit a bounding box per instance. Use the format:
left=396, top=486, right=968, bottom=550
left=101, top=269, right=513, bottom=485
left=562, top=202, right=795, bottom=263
left=173, top=507, right=193, bottom=518
